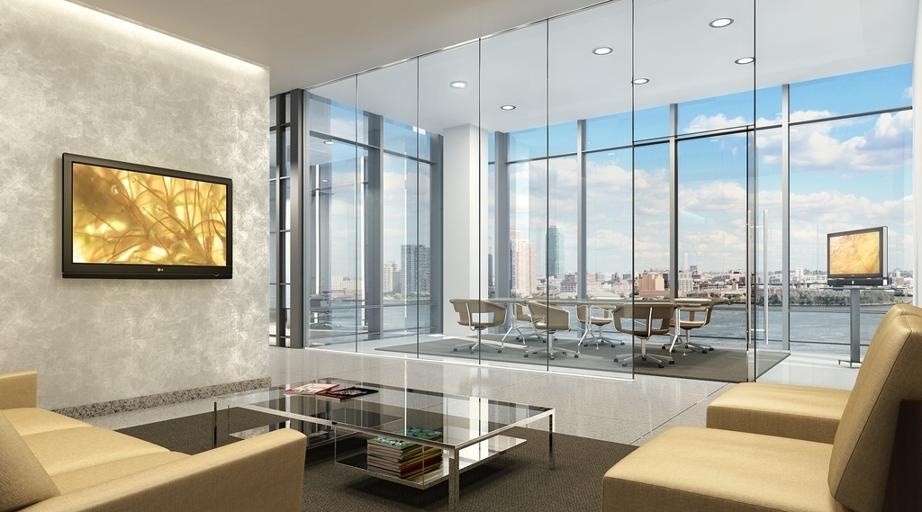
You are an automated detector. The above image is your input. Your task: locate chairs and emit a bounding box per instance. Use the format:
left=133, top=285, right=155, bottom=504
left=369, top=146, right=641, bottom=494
left=600, top=315, right=922, bottom=512
left=448, top=298, right=713, bottom=369
left=706, top=304, right=922, bottom=444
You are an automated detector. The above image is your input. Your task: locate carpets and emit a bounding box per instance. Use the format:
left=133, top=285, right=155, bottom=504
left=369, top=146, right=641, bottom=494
left=374, top=333, right=791, bottom=383
left=112, top=395, right=640, bottom=512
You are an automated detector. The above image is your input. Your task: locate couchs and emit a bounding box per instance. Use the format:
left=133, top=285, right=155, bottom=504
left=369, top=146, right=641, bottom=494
left=0, top=370, right=307, bottom=512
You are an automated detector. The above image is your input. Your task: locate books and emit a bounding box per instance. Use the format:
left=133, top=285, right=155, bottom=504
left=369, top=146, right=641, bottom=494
left=283, top=383, right=339, bottom=396
left=315, top=387, right=378, bottom=400
left=367, top=426, right=443, bottom=480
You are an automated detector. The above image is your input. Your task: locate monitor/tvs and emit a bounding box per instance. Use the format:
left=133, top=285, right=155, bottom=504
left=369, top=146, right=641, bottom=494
left=827, top=226, right=888, bottom=286
left=62, top=153, right=233, bottom=279
